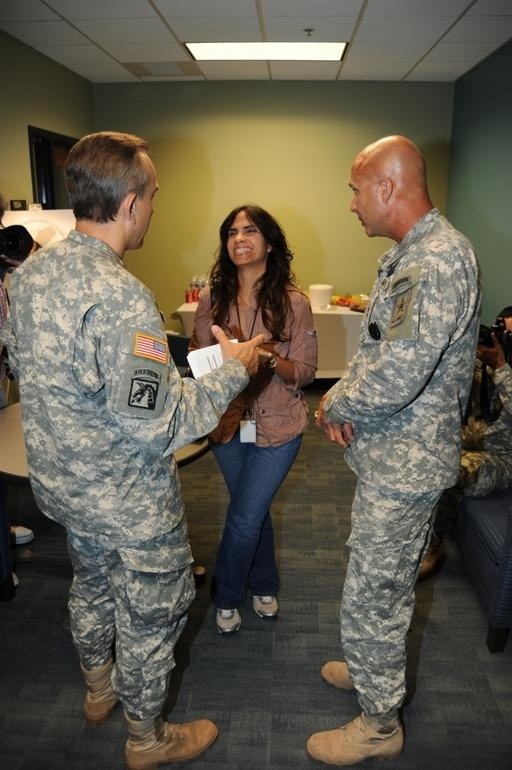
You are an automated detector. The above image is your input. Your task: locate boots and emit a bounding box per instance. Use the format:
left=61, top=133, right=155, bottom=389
left=124, top=704, right=218, bottom=770
left=418, top=542, right=451, bottom=581
left=79, top=656, right=118, bottom=724
left=306, top=711, right=404, bottom=766
left=321, top=661, right=354, bottom=690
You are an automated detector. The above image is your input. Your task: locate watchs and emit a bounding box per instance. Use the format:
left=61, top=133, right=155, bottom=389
left=264, top=353, right=278, bottom=368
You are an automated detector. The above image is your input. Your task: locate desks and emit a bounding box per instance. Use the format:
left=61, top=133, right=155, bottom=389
left=176, top=302, right=365, bottom=379
left=0, top=400, right=211, bottom=631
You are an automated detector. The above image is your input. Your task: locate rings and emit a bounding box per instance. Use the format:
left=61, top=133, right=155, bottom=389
left=314, top=410, right=319, bottom=417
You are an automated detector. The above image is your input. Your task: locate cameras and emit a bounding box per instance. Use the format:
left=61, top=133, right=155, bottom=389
left=478, top=316, right=512, bottom=353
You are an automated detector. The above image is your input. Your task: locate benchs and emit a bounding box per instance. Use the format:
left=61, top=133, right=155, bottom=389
left=461, top=493, right=512, bottom=656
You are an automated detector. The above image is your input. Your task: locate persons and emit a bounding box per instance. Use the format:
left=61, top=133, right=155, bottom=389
left=0, top=226, right=33, bottom=543
left=0, top=131, right=271, bottom=767
left=187, top=206, right=317, bottom=632
left=308, top=132, right=484, bottom=767
left=419, top=305, right=511, bottom=572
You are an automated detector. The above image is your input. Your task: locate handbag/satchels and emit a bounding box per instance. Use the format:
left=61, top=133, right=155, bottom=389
left=207, top=396, right=247, bottom=445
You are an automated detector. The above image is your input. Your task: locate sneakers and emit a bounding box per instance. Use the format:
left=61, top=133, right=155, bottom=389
left=216, top=607, right=241, bottom=634
left=252, top=594, right=279, bottom=618
left=9, top=524, right=34, bottom=545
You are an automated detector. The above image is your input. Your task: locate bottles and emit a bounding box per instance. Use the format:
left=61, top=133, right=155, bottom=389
left=189, top=274, right=209, bottom=288
